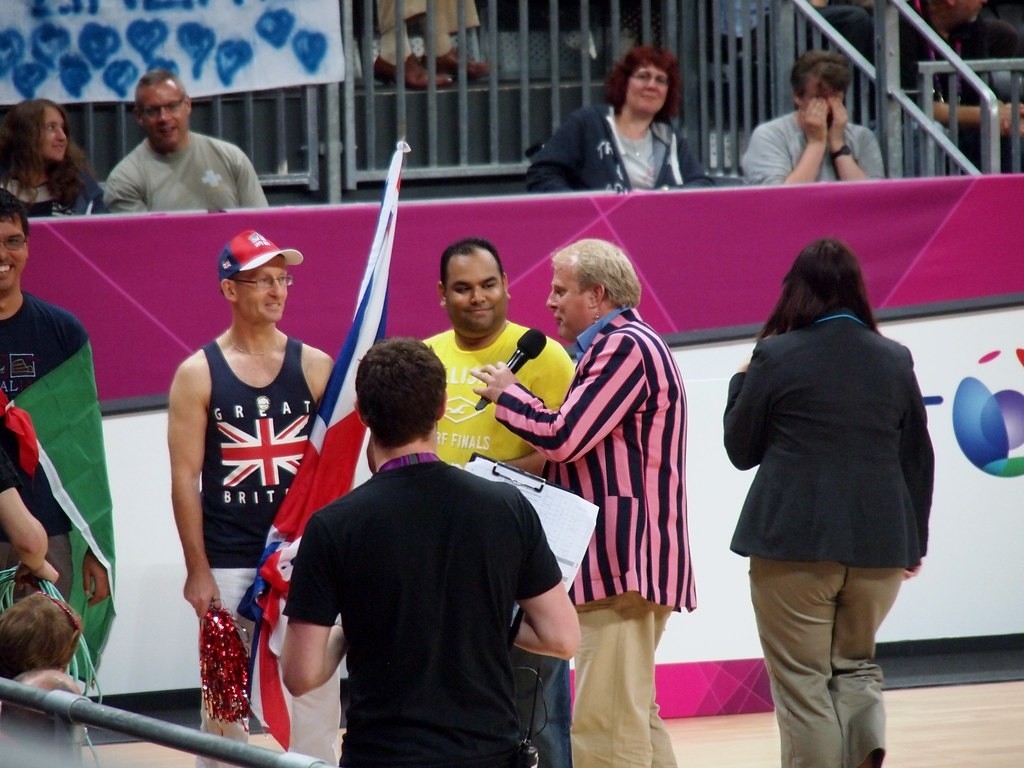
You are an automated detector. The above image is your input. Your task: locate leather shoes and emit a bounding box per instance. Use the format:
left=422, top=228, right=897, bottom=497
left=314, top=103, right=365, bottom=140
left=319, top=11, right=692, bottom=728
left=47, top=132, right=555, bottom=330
left=374, top=53, right=452, bottom=89
left=418, top=48, right=493, bottom=82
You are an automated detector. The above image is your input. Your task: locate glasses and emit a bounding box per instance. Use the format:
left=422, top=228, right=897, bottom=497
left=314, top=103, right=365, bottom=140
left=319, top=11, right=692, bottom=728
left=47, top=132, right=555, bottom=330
left=36, top=588, right=82, bottom=659
left=137, top=92, right=187, bottom=120
left=230, top=275, right=294, bottom=288
left=0, top=231, right=27, bottom=251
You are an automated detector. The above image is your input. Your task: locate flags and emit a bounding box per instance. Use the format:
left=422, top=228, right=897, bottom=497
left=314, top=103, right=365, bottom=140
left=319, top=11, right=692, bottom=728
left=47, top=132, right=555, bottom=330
left=235, top=142, right=410, bottom=756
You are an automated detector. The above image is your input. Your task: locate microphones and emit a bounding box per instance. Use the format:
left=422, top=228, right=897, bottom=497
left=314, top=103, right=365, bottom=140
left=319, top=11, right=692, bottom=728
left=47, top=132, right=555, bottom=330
left=475, top=328, right=547, bottom=410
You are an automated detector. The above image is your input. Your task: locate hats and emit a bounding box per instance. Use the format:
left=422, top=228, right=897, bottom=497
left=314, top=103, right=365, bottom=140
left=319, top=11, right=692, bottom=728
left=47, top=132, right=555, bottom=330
left=217, top=230, right=303, bottom=281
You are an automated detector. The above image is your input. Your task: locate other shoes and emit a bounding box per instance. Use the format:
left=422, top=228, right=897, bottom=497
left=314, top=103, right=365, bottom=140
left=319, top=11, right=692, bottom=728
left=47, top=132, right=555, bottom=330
left=855, top=749, right=885, bottom=768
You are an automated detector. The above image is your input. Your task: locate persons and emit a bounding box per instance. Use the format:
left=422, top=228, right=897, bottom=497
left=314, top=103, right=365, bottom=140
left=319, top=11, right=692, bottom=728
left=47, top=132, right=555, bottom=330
left=423, top=237, right=696, bottom=768
left=739, top=52, right=885, bottom=186
left=0, top=97, right=109, bottom=215
left=810, top=0, right=1024, bottom=175
left=172, top=229, right=342, bottom=768
left=0, top=187, right=116, bottom=768
left=104, top=69, right=269, bottom=214
left=277, top=337, right=580, bottom=768
left=722, top=236, right=935, bottom=768
left=528, top=47, right=713, bottom=195
left=374, top=0, right=492, bottom=88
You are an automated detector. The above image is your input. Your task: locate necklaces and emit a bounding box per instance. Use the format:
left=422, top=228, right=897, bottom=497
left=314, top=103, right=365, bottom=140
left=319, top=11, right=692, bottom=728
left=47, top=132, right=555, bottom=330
left=227, top=329, right=279, bottom=355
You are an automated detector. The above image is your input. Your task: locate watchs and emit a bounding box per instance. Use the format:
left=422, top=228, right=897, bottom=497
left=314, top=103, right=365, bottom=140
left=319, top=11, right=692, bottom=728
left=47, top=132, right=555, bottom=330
left=832, top=145, right=851, bottom=158
